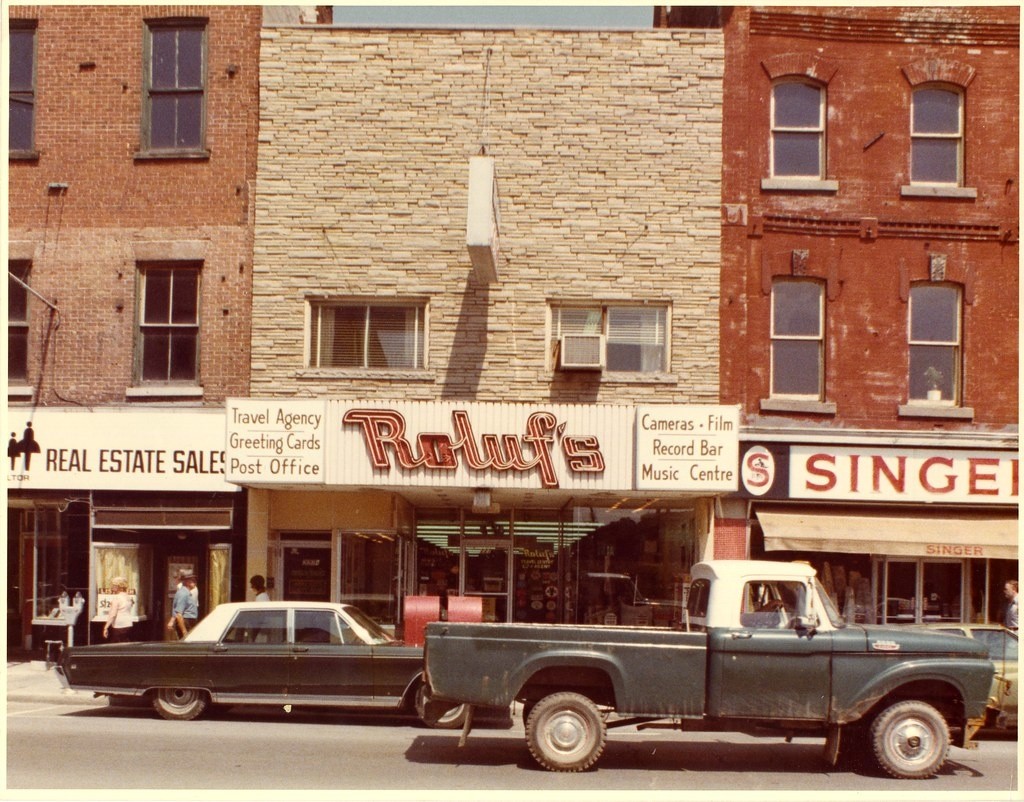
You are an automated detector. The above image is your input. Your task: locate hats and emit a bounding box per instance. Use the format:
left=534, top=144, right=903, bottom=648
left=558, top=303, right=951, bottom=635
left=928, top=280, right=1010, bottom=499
left=179, top=570, right=198, bottom=579
left=112, top=577, right=128, bottom=586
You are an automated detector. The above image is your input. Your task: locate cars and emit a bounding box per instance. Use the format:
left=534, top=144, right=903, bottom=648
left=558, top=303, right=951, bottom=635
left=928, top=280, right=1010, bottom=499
left=905, top=623, right=1018, bottom=733
left=56, top=602, right=511, bottom=729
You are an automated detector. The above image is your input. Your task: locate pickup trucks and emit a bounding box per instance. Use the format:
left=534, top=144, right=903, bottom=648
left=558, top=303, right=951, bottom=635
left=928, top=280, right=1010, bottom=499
left=588, top=572, right=659, bottom=628
left=420, top=559, right=996, bottom=779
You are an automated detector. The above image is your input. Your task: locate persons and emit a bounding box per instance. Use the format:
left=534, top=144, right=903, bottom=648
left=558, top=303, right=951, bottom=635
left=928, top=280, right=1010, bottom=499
left=1004, top=579, right=1018, bottom=637
left=103, top=576, right=136, bottom=645
left=166, top=568, right=201, bottom=640
left=742, top=594, right=785, bottom=627
left=250, top=575, right=270, bottom=603
